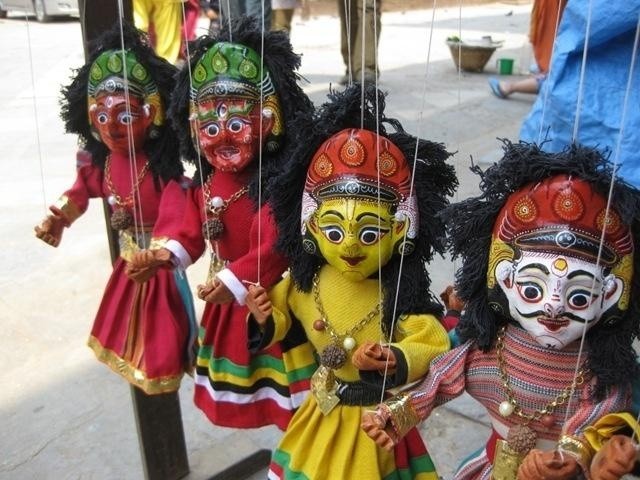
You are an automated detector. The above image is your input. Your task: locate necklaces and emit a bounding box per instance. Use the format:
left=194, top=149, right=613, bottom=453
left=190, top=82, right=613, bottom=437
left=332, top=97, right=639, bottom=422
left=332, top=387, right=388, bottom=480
left=494, top=324, right=592, bottom=452
left=309, top=262, right=383, bottom=370
left=202, top=169, right=251, bottom=240
left=104, top=154, right=152, bottom=231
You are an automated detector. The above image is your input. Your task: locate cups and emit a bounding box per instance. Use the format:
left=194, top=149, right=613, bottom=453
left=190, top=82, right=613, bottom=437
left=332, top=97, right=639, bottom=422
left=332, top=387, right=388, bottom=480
left=496, top=58, right=514, bottom=75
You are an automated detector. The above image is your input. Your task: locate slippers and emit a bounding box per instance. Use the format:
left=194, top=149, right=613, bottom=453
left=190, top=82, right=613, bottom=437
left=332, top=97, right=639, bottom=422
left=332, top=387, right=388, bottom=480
left=488, top=76, right=506, bottom=98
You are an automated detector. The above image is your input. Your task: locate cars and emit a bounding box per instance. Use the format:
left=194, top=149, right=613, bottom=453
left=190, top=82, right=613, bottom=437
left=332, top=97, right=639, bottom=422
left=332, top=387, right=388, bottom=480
left=0, top=2, right=80, bottom=21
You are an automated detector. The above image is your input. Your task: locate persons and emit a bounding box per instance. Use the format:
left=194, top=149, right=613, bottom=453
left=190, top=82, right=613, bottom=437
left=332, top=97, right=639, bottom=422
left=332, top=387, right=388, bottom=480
left=336, top=0, right=384, bottom=86
left=243, top=81, right=453, bottom=480
left=131, top=0, right=298, bottom=66
left=125, top=12, right=316, bottom=433
left=34, top=20, right=197, bottom=396
left=360, top=124, right=640, bottom=480
left=487, top=71, right=548, bottom=99
left=582, top=411, right=640, bottom=480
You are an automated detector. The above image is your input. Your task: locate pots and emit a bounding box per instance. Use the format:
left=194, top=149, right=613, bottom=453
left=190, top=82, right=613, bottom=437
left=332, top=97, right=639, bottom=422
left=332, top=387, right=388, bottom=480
left=445, top=41, right=496, bottom=73
